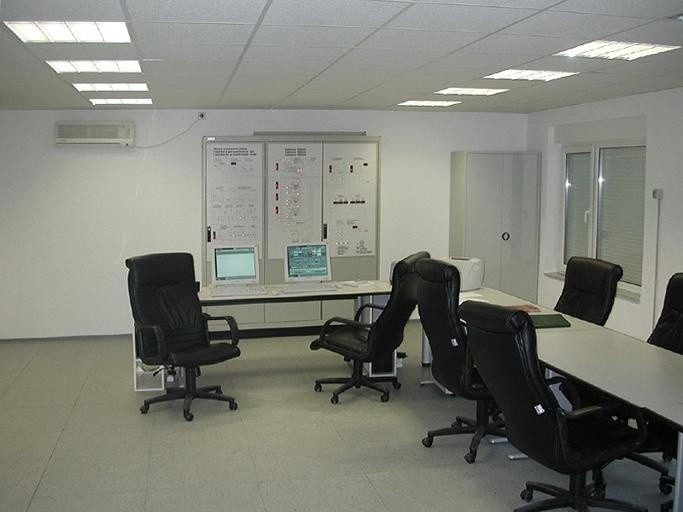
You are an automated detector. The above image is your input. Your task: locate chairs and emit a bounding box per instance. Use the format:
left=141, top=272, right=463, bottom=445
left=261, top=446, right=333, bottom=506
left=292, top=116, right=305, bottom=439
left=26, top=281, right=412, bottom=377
left=310, top=251, right=432, bottom=403
left=648, top=272, right=681, bottom=354
left=554, top=255, right=624, bottom=330
left=459, top=299, right=646, bottom=512
left=405, top=257, right=515, bottom=463
left=126, top=253, right=241, bottom=420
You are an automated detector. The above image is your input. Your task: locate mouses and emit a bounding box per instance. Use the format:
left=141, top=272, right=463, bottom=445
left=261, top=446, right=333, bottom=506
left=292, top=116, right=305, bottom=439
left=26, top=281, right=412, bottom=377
left=272, top=288, right=279, bottom=295
left=336, top=283, right=343, bottom=288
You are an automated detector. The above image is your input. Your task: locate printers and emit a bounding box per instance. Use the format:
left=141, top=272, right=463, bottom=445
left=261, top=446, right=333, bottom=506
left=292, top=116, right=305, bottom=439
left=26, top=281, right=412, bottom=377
left=440, top=256, right=485, bottom=291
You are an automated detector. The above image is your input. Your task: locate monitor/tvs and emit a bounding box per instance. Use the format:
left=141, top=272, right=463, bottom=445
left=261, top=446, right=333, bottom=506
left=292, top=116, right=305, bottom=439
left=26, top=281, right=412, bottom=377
left=283, top=243, right=332, bottom=282
left=211, top=245, right=260, bottom=285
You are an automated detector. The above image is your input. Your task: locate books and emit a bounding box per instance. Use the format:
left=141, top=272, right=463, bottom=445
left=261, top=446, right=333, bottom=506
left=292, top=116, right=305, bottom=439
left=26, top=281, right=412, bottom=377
left=341, top=278, right=374, bottom=287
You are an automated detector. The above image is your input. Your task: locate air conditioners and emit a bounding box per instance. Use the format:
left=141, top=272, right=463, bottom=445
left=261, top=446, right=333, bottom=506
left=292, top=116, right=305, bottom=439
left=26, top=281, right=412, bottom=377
left=54, top=121, right=135, bottom=148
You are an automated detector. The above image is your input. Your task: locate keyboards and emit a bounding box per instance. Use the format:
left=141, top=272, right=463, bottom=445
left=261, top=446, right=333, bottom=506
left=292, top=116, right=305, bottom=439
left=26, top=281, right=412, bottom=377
left=280, top=284, right=336, bottom=293
left=211, top=287, right=268, bottom=297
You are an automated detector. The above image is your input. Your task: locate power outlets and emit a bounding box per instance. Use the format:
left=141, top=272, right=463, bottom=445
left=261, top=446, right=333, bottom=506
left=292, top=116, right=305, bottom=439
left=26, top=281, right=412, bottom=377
left=198, top=112, right=206, bottom=120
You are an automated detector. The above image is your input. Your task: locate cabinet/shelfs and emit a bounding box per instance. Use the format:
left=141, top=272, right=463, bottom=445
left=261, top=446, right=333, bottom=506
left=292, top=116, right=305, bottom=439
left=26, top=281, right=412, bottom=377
left=450, top=150, right=542, bottom=305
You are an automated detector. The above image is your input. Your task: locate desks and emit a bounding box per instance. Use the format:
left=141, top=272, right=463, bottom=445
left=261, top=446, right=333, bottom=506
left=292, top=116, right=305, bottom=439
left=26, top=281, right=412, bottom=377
left=456, top=287, right=682, bottom=512
left=199, top=278, right=397, bottom=379
